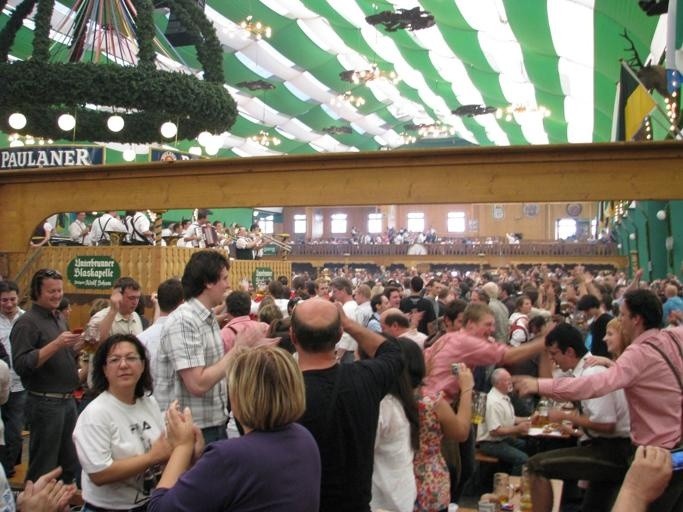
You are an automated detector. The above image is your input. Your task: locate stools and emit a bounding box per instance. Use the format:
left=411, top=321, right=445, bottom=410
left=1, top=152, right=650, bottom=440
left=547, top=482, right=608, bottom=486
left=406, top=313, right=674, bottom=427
left=474, top=451, right=500, bottom=491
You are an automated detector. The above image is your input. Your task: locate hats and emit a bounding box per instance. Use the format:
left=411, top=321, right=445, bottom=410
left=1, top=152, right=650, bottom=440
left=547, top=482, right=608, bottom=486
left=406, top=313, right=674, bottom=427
left=481, top=281, right=497, bottom=298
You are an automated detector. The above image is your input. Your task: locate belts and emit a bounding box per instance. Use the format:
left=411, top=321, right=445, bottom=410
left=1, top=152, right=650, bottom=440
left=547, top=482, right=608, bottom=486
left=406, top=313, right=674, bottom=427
left=27, top=390, right=75, bottom=401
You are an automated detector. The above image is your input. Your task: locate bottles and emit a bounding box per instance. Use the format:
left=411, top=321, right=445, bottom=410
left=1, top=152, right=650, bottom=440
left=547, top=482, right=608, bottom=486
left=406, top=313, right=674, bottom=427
left=537, top=396, right=548, bottom=426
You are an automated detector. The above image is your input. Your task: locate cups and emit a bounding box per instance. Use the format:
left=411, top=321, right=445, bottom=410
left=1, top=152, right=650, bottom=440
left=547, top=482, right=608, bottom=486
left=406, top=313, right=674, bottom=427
left=494, top=473, right=514, bottom=501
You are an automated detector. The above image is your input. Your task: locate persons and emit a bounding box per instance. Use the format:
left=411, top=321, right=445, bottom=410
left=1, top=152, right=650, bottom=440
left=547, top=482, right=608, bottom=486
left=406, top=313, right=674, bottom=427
left=527, top=324, right=630, bottom=512
left=400, top=276, right=436, bottom=336
left=269, top=281, right=290, bottom=318
left=58, top=299, right=70, bottom=329
left=355, top=284, right=373, bottom=327
left=510, top=293, right=534, bottom=347
left=29, top=217, right=52, bottom=248
left=259, top=294, right=275, bottom=309
left=234, top=226, right=259, bottom=261
left=612, top=445, right=672, bottom=512
left=307, top=225, right=522, bottom=245
left=384, top=288, right=400, bottom=308
left=89, top=210, right=127, bottom=246
left=248, top=281, right=263, bottom=320
left=398, top=337, right=474, bottom=512
left=662, top=285, right=683, bottom=326
left=278, top=276, right=291, bottom=299
left=248, top=224, right=271, bottom=259
left=221, top=292, right=270, bottom=354
left=10, top=268, right=85, bottom=485
left=237, top=277, right=249, bottom=293
left=78, top=298, right=109, bottom=384
left=154, top=251, right=282, bottom=445
left=173, top=224, right=185, bottom=236
left=164, top=223, right=175, bottom=236
left=522, top=287, right=560, bottom=341
left=422, top=303, right=556, bottom=512
left=72, top=334, right=173, bottom=512
left=367, top=294, right=391, bottom=333
left=424, top=280, right=441, bottom=329
left=484, top=283, right=509, bottom=345
left=471, top=288, right=489, bottom=306
left=0, top=280, right=27, bottom=477
left=332, top=277, right=364, bottom=365
left=135, top=279, right=185, bottom=388
left=477, top=368, right=532, bottom=477
left=513, top=289, right=683, bottom=512
left=0, top=463, right=77, bottom=512
left=85, top=277, right=143, bottom=390
left=129, top=210, right=156, bottom=245
left=147, top=346, right=322, bottom=512
left=1, top=360, right=10, bottom=446
left=213, top=221, right=231, bottom=258
left=576, top=295, right=617, bottom=362
left=361, top=265, right=491, bottom=292
left=181, top=220, right=192, bottom=235
left=585, top=295, right=613, bottom=351
left=583, top=317, right=631, bottom=368
left=120, top=210, right=136, bottom=246
left=258, top=305, right=281, bottom=328
left=266, top=318, right=296, bottom=355
left=498, top=282, right=516, bottom=318
left=288, top=266, right=361, bottom=316
left=381, top=307, right=428, bottom=349
left=369, top=333, right=420, bottom=512
left=437, top=285, right=450, bottom=325
left=538, top=261, right=683, bottom=295
left=136, top=292, right=159, bottom=331
left=233, top=297, right=402, bottom=512
left=184, top=209, right=208, bottom=248
left=214, top=290, right=233, bottom=326
left=426, top=301, right=467, bottom=349
left=68, top=212, right=89, bottom=245
left=500, top=263, right=538, bottom=289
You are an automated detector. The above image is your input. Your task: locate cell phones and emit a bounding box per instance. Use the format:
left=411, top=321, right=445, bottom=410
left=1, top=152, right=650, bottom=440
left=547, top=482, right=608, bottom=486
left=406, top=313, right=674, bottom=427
left=72, top=328, right=84, bottom=334
left=670, top=448, right=683, bottom=471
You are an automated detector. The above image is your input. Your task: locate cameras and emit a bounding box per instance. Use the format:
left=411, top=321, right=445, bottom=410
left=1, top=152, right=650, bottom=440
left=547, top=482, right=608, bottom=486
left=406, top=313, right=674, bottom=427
left=451, top=364, right=458, bottom=375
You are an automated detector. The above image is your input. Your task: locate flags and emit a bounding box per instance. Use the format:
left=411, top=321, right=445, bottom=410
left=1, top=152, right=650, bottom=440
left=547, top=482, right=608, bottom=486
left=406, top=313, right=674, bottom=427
left=615, top=57, right=656, bottom=142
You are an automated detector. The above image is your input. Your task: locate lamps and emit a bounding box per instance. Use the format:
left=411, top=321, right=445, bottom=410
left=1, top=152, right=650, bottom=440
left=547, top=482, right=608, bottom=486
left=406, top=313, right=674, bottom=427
left=227, top=14, right=272, bottom=43
left=617, top=243, right=622, bottom=249
left=8, top=112, right=27, bottom=130
left=656, top=209, right=667, bottom=221
left=107, top=115, right=124, bottom=132
left=247, top=130, right=281, bottom=147
left=628, top=233, right=636, bottom=240
left=160, top=121, right=178, bottom=138
left=57, top=113, right=75, bottom=131
